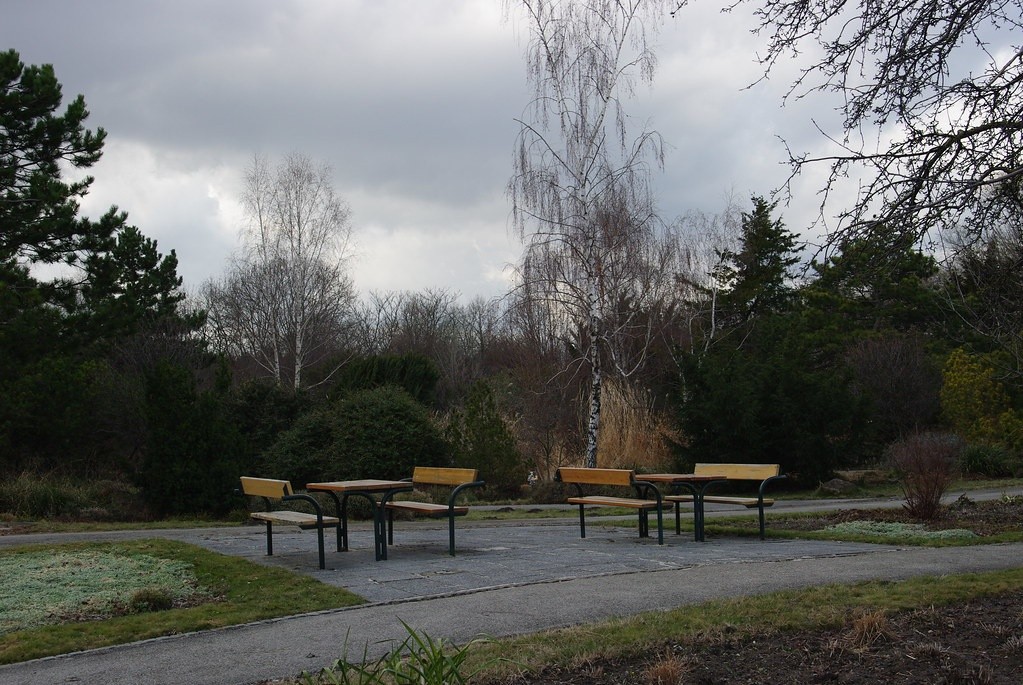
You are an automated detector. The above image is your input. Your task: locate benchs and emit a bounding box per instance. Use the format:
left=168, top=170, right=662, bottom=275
left=376, top=466, right=484, bottom=557
left=664, top=463, right=786, bottom=540
left=555, top=468, right=663, bottom=545
left=240, top=476, right=340, bottom=569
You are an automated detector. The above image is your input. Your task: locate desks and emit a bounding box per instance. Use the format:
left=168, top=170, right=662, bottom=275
left=634, top=474, right=727, bottom=542
left=306, top=479, right=413, bottom=562
